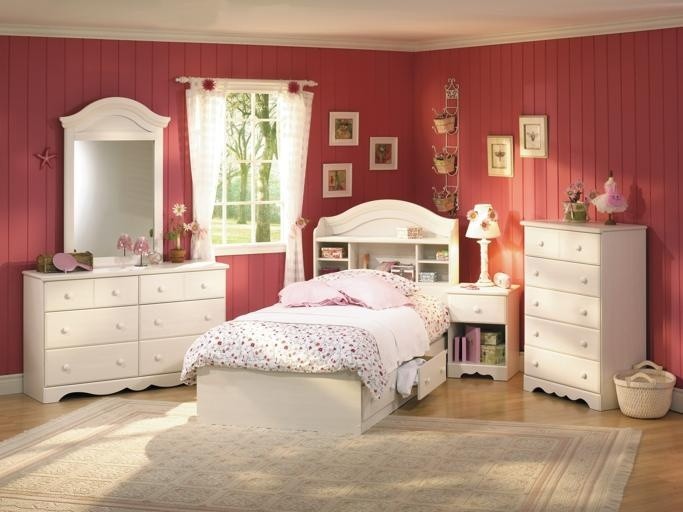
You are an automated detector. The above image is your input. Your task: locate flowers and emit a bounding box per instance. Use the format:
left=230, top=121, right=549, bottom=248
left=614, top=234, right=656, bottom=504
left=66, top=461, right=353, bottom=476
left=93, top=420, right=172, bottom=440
left=566, top=179, right=599, bottom=204
left=168, top=201, right=208, bottom=249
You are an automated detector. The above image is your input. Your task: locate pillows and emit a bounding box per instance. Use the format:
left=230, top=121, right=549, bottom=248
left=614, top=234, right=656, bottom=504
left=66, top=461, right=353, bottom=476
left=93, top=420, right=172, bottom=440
left=277, top=281, right=349, bottom=307
left=328, top=272, right=415, bottom=310
left=309, top=269, right=421, bottom=296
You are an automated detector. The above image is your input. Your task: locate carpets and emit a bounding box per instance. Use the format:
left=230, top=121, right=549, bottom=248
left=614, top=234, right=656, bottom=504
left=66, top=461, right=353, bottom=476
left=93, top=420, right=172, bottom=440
left=0, top=397, right=644, bottom=512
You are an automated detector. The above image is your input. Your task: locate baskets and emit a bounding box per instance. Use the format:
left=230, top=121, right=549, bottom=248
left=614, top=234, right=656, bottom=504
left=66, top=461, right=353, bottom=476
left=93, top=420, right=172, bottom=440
left=613, top=361, right=677, bottom=419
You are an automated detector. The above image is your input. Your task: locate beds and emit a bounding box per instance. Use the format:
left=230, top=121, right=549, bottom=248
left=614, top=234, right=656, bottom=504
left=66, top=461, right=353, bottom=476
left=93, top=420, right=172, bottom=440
left=197, top=198, right=459, bottom=435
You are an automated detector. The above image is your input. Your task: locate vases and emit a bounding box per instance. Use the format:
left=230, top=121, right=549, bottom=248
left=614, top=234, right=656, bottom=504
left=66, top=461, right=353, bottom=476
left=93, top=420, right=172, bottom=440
left=170, top=249, right=186, bottom=263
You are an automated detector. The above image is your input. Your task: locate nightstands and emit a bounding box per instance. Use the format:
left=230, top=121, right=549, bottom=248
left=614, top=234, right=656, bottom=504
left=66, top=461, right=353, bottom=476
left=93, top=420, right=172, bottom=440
left=447, top=283, right=521, bottom=382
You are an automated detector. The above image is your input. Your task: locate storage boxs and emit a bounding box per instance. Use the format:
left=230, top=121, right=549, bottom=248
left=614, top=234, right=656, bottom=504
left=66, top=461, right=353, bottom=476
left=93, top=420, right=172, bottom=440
left=481, top=345, right=506, bottom=366
left=481, top=332, right=503, bottom=345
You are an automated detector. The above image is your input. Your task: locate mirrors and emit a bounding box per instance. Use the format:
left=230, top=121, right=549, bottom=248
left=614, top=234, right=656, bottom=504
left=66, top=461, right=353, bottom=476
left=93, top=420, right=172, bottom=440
left=59, top=97, right=171, bottom=266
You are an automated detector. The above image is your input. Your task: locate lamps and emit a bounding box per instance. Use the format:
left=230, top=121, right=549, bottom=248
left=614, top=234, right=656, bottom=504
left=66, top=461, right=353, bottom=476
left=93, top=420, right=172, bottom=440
left=465, top=202, right=502, bottom=286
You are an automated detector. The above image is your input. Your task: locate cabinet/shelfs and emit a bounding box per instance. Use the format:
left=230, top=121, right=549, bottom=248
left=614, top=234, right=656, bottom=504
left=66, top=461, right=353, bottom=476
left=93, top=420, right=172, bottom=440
left=521, top=219, right=648, bottom=411
left=21, top=260, right=230, bottom=404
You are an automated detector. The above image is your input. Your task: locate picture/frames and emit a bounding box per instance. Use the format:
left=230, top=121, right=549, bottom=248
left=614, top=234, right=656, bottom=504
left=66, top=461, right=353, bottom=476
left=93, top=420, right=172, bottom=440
left=370, top=137, right=397, bottom=170
left=518, top=115, right=548, bottom=158
left=329, top=112, right=360, bottom=146
left=322, top=163, right=352, bottom=198
left=486, top=136, right=514, bottom=177
